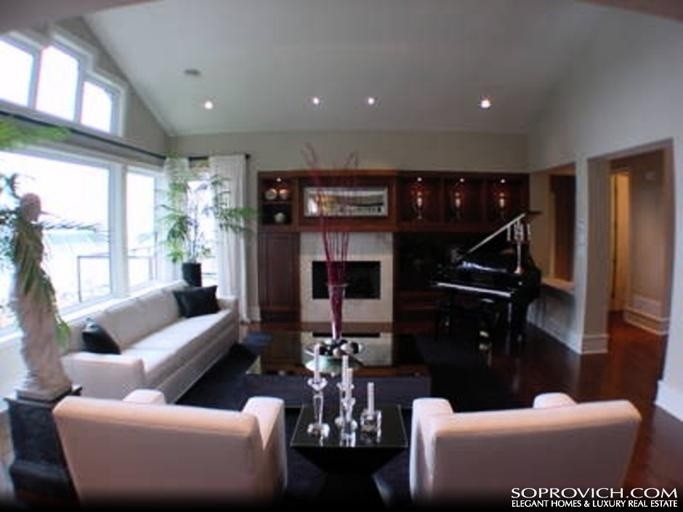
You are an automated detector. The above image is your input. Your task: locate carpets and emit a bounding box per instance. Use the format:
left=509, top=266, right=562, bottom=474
left=198, top=372, right=431, bottom=502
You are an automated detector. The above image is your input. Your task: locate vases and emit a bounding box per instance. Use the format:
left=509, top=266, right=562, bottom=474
left=327, top=284, right=347, bottom=339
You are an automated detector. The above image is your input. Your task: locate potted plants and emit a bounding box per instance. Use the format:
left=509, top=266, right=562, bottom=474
left=152, top=166, right=258, bottom=286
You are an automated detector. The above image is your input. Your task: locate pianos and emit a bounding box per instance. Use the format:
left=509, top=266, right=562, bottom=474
left=429, top=211, right=543, bottom=353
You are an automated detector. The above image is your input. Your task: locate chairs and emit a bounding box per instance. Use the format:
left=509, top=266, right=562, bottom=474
left=56, top=393, right=288, bottom=507
left=408, top=391, right=642, bottom=505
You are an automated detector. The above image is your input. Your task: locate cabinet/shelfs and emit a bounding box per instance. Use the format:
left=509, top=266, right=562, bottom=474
left=259, top=169, right=529, bottom=323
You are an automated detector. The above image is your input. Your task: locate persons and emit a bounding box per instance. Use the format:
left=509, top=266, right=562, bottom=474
left=6, top=192, right=69, bottom=390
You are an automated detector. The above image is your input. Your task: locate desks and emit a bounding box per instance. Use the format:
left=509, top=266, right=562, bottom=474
left=287, top=402, right=409, bottom=512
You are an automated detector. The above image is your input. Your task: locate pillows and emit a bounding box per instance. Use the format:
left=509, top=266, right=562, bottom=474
left=81, top=316, right=122, bottom=353
left=173, top=285, right=219, bottom=317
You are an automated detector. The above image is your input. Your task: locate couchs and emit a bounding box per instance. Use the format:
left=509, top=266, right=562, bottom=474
left=60, top=288, right=240, bottom=402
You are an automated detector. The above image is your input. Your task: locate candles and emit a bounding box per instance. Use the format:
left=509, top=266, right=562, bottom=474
left=341, top=355, right=349, bottom=385
left=345, top=368, right=353, bottom=403
left=314, top=345, right=321, bottom=385
left=365, top=382, right=376, bottom=415
